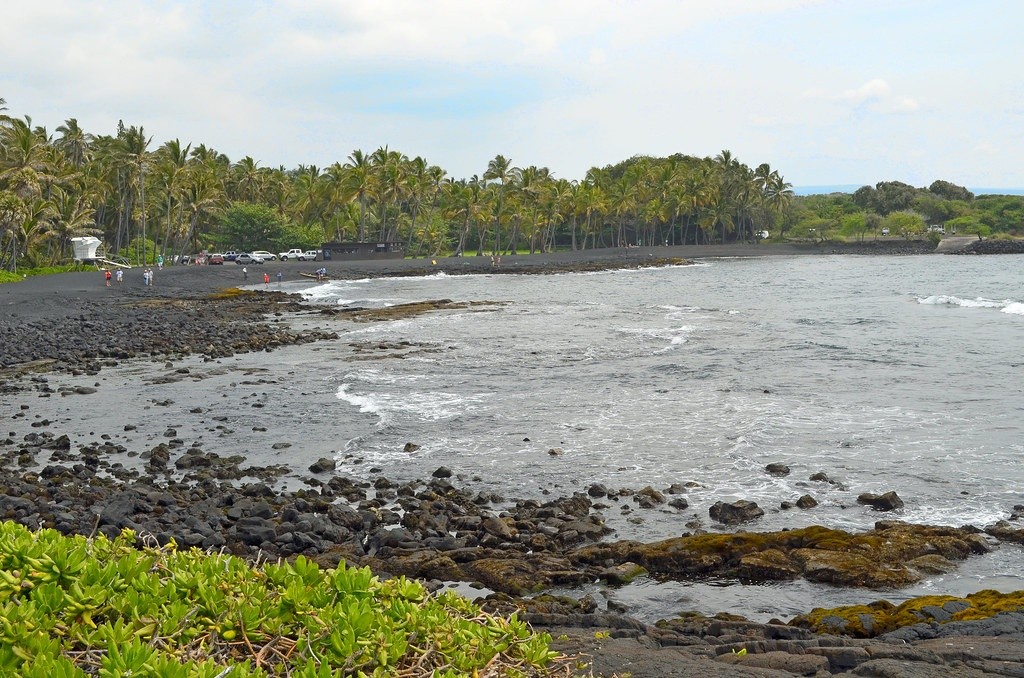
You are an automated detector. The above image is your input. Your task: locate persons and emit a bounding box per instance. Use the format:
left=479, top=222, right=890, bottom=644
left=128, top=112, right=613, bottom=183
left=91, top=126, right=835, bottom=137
left=147, top=267, right=153, bottom=286
left=116, top=268, right=123, bottom=285
left=105, top=269, right=112, bottom=287
left=316, top=267, right=326, bottom=280
left=157, top=254, right=163, bottom=271
left=495, top=256, right=501, bottom=268
left=243, top=266, right=247, bottom=280
left=276, top=271, right=285, bottom=287
left=624, top=245, right=629, bottom=256
left=490, top=255, right=495, bottom=267
left=263, top=273, right=270, bottom=289
left=143, top=269, right=149, bottom=286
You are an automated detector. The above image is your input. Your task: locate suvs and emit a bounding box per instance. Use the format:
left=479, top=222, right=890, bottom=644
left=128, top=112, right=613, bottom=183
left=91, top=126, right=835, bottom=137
left=251, top=250, right=277, bottom=262
left=278, top=248, right=304, bottom=262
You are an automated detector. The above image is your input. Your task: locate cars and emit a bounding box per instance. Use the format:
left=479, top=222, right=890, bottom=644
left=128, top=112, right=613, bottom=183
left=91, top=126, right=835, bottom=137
left=221, top=250, right=240, bottom=260
left=233, top=252, right=264, bottom=265
left=170, top=251, right=224, bottom=267
left=296, top=251, right=320, bottom=261
